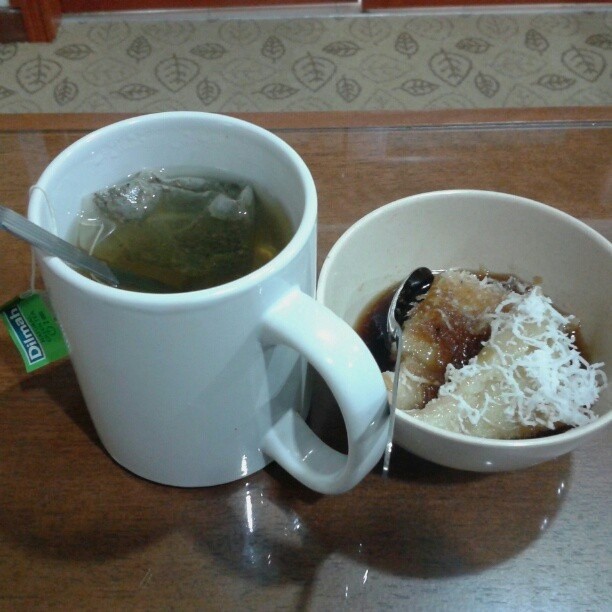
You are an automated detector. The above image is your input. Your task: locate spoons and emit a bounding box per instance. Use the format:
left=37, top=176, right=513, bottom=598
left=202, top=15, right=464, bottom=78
left=382, top=267, right=433, bottom=471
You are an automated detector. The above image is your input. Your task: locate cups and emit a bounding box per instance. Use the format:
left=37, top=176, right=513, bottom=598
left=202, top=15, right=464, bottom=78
left=25, top=111, right=389, bottom=497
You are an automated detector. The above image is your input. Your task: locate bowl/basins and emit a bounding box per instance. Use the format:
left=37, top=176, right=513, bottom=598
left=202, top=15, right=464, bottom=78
left=317, top=189, right=612, bottom=472
